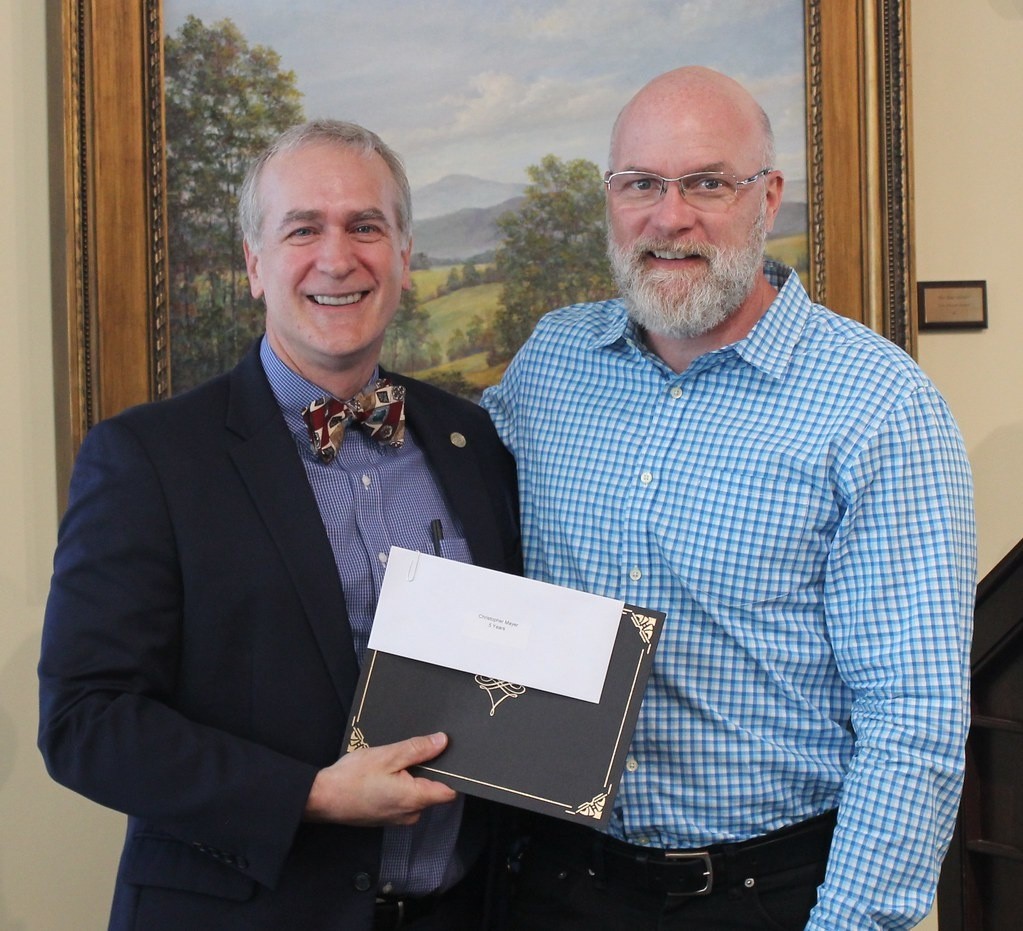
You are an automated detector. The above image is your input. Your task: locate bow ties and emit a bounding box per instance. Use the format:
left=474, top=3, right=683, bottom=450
left=301, top=378, right=406, bottom=465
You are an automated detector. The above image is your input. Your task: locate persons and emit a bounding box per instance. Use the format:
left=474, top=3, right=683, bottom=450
left=477, top=64, right=977, bottom=931
left=36, top=119, right=521, bottom=931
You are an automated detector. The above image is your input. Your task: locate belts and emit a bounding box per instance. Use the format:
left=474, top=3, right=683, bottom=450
left=379, top=892, right=441, bottom=931
left=540, top=823, right=842, bottom=898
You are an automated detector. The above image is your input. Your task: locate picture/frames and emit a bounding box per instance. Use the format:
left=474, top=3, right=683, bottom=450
left=46, top=0, right=918, bottom=466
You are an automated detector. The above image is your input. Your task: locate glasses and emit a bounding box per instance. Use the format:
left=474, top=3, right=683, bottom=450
left=602, top=167, right=773, bottom=213
